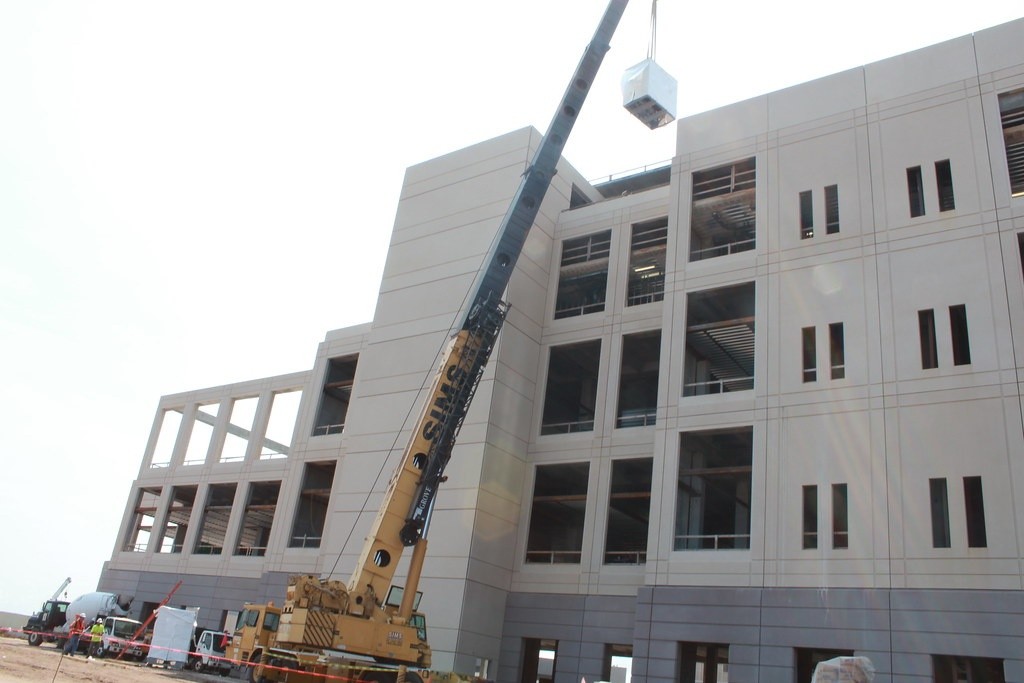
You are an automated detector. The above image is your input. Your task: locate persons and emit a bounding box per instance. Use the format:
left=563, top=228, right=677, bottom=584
left=87, top=618, right=105, bottom=658
left=62, top=612, right=86, bottom=657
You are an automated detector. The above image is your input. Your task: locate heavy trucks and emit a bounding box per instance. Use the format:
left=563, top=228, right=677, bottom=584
left=143, top=624, right=235, bottom=674
left=22, top=577, right=143, bottom=660
left=220, top=0, right=679, bottom=683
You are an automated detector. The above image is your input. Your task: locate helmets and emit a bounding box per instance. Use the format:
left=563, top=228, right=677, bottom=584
left=80, top=613, right=86, bottom=617
left=97, top=618, right=103, bottom=623
